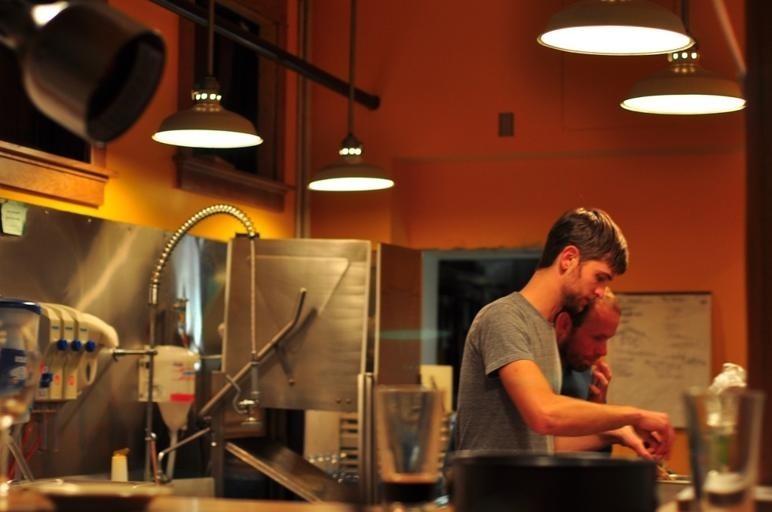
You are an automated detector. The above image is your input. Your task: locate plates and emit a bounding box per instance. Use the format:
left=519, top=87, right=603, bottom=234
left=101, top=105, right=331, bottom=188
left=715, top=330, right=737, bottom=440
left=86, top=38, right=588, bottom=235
left=26, top=480, right=176, bottom=512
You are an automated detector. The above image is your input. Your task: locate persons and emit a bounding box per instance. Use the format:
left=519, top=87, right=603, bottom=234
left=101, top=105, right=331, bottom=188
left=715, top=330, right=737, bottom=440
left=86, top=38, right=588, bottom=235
left=455, top=207, right=676, bottom=464
left=554, top=288, right=621, bottom=456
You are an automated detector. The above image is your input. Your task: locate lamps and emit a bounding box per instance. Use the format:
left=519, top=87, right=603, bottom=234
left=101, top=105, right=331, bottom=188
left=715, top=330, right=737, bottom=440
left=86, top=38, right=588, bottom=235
left=619, top=0, right=748, bottom=116
left=306, top=0, right=395, bottom=192
left=150, top=0, right=264, bottom=149
left=536, top=0, right=696, bottom=57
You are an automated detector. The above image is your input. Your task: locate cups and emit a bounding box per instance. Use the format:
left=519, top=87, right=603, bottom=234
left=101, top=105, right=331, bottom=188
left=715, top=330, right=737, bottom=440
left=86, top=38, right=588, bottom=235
left=0, top=298, right=43, bottom=424
left=372, top=384, right=450, bottom=512
left=678, top=387, right=769, bottom=512
